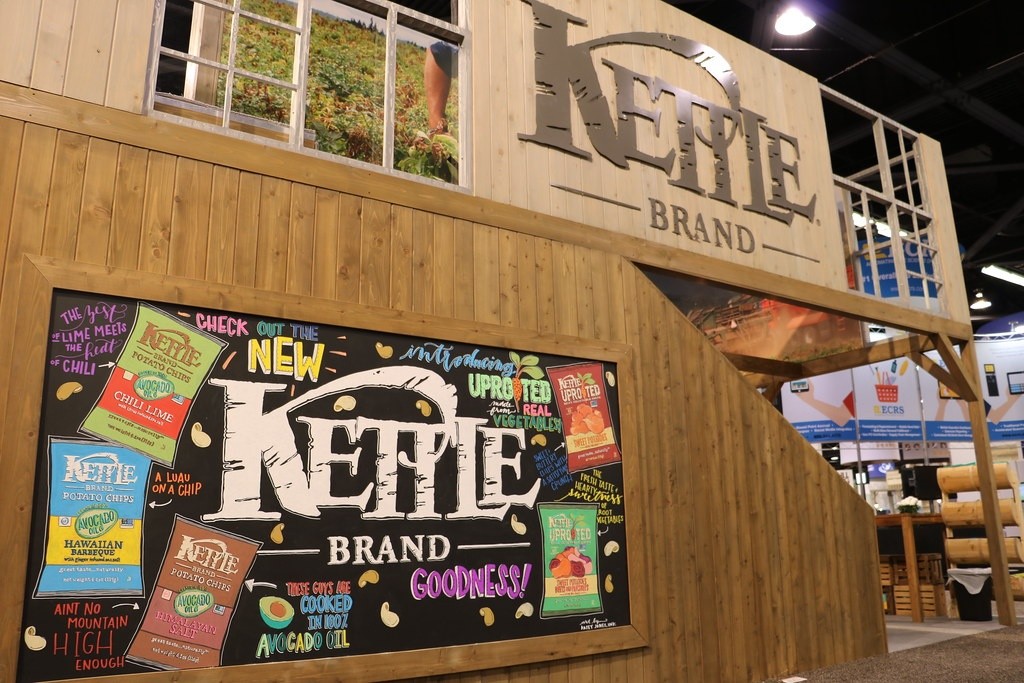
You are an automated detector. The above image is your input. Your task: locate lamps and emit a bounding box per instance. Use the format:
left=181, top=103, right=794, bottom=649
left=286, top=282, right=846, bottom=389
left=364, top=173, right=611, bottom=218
left=969, top=290, right=992, bottom=309
left=980, top=263, right=1024, bottom=286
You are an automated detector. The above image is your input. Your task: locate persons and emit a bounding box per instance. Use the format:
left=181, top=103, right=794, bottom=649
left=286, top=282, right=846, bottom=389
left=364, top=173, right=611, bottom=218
left=422, top=40, right=460, bottom=157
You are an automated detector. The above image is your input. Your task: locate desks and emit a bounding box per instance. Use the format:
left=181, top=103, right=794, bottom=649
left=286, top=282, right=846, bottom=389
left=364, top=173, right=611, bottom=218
left=875, top=514, right=960, bottom=623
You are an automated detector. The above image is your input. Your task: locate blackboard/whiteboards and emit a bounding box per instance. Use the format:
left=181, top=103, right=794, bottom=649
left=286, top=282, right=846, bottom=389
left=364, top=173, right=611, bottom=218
left=0, top=265, right=651, bottom=683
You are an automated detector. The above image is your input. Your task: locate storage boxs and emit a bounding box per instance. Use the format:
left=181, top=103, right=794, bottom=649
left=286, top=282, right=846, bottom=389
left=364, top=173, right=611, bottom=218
left=880, top=554, right=948, bottom=617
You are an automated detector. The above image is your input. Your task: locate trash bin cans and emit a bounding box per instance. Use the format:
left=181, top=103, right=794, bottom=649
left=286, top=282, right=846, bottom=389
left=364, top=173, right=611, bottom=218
left=882, top=582, right=897, bottom=615
left=946, top=565, right=993, bottom=623
